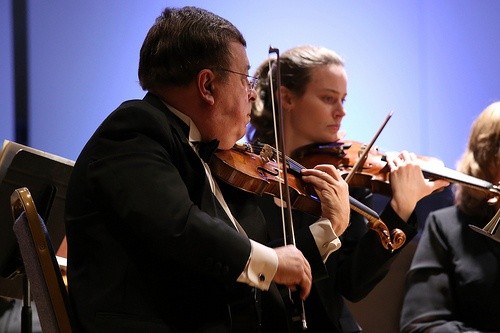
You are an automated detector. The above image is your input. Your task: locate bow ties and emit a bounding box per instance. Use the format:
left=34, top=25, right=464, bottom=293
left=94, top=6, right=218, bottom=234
left=196, top=138, right=219, bottom=162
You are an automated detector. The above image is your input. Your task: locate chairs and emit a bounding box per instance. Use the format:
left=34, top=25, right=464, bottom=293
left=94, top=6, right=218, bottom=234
left=9, top=187, right=75, bottom=333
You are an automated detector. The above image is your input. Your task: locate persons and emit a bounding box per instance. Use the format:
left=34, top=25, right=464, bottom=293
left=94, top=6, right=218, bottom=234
left=249, top=45, right=449, bottom=333
left=396, top=101, right=499, bottom=332
left=65, top=7, right=350, bottom=332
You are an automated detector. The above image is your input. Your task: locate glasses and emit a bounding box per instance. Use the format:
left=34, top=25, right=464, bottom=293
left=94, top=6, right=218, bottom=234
left=213, top=67, right=258, bottom=92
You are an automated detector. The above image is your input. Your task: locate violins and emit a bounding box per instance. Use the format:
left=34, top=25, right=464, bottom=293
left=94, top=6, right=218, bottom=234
left=207, top=135, right=406, bottom=252
left=289, top=134, right=500, bottom=205
